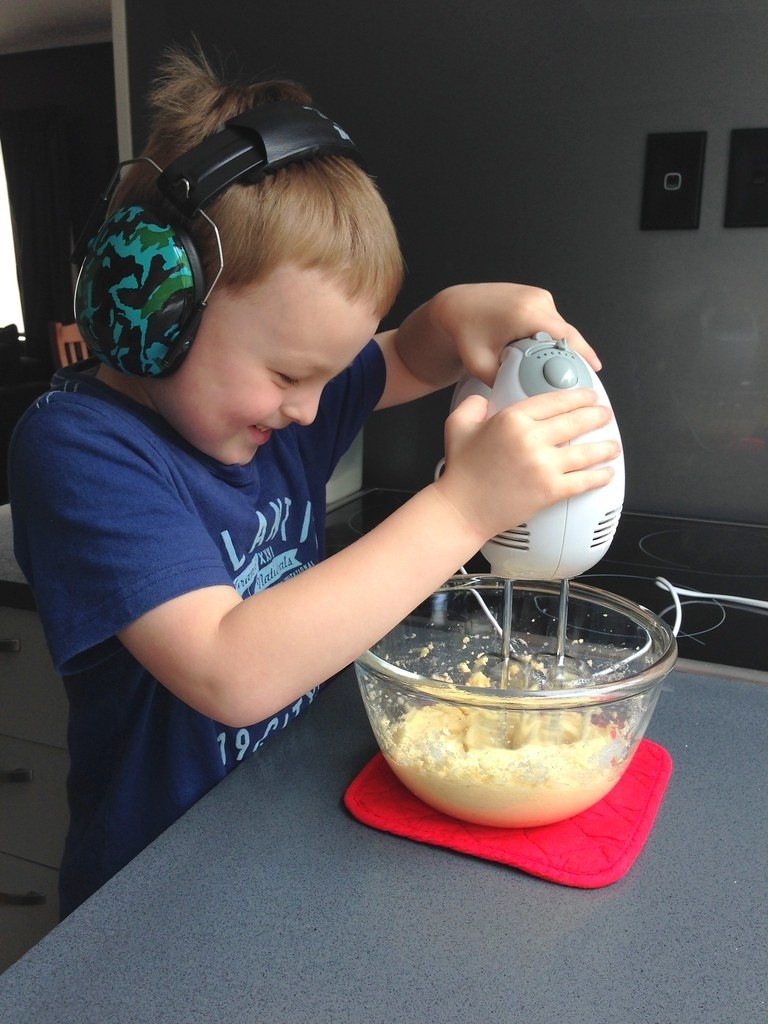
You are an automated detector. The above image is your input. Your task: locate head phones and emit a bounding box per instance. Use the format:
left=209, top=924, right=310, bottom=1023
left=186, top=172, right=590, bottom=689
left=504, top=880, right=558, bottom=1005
left=70, top=100, right=367, bottom=382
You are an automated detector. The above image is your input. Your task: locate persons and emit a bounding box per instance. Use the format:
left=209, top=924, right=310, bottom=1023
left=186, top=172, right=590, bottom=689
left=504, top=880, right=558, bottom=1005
left=8, top=56, right=622, bottom=920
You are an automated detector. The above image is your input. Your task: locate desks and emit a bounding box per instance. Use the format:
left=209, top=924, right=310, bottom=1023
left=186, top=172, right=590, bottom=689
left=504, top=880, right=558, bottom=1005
left=0, top=617, right=768, bottom=1024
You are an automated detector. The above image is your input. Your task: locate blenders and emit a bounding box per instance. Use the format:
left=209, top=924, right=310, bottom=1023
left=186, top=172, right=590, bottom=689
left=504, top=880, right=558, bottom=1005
left=446, top=331, right=626, bottom=750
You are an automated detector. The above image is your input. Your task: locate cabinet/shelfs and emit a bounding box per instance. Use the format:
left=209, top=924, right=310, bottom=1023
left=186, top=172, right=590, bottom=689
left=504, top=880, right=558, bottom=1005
left=0, top=506, right=69, bottom=966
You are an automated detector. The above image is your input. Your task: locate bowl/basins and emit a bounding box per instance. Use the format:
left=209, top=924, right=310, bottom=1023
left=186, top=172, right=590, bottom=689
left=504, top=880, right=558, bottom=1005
left=351, top=574, right=679, bottom=831
